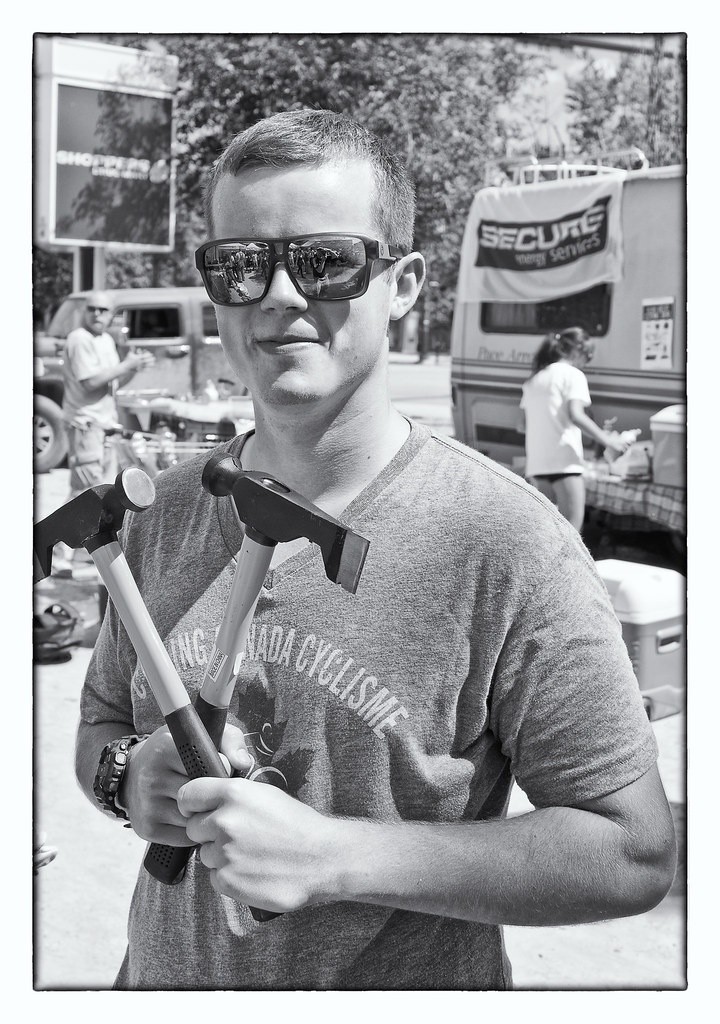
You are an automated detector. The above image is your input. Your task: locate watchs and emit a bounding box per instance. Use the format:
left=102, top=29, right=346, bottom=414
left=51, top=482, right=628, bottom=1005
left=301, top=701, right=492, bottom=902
left=94, top=735, right=152, bottom=828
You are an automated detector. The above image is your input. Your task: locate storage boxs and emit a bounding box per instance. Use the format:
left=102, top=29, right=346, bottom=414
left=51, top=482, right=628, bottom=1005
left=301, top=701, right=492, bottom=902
left=647, top=404, right=684, bottom=489
left=585, top=561, right=685, bottom=722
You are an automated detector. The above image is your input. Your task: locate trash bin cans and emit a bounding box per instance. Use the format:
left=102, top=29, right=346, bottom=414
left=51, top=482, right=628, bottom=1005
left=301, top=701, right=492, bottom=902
left=593, top=558, right=686, bottom=723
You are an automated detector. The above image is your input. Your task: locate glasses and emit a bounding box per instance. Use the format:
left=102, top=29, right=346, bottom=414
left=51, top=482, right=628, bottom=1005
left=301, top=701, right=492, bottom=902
left=87, top=305, right=109, bottom=314
left=195, top=232, right=405, bottom=307
left=585, top=353, right=593, bottom=363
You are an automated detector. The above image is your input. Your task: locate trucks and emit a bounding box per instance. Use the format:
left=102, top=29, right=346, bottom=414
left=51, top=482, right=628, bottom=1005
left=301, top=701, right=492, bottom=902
left=447, top=162, right=686, bottom=468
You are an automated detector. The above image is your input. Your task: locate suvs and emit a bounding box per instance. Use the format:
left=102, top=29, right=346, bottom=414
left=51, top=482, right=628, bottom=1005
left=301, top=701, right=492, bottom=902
left=32, top=286, right=251, bottom=475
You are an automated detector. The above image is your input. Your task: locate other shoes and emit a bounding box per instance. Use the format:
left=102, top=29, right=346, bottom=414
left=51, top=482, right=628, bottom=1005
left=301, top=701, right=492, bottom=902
left=237, top=281, right=242, bottom=283
left=242, top=279, right=244, bottom=281
left=297, top=273, right=300, bottom=275
left=302, top=276, right=306, bottom=278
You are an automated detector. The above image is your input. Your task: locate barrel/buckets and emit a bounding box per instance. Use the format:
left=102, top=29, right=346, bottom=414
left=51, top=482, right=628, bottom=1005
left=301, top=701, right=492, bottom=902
left=649, top=403, right=687, bottom=487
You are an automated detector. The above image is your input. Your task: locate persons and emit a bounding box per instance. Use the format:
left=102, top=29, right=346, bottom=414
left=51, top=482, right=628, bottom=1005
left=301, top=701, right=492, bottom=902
left=74, top=106, right=680, bottom=991
left=61, top=291, right=157, bottom=502
left=517, top=325, right=637, bottom=539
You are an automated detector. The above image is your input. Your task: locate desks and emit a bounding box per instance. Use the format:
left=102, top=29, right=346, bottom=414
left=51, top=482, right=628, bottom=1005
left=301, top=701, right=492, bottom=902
left=515, top=447, right=688, bottom=558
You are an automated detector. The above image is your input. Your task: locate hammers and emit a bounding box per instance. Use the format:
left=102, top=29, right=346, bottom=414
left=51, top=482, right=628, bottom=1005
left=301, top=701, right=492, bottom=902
left=141, top=454, right=371, bottom=886
left=34, top=466, right=284, bottom=922
